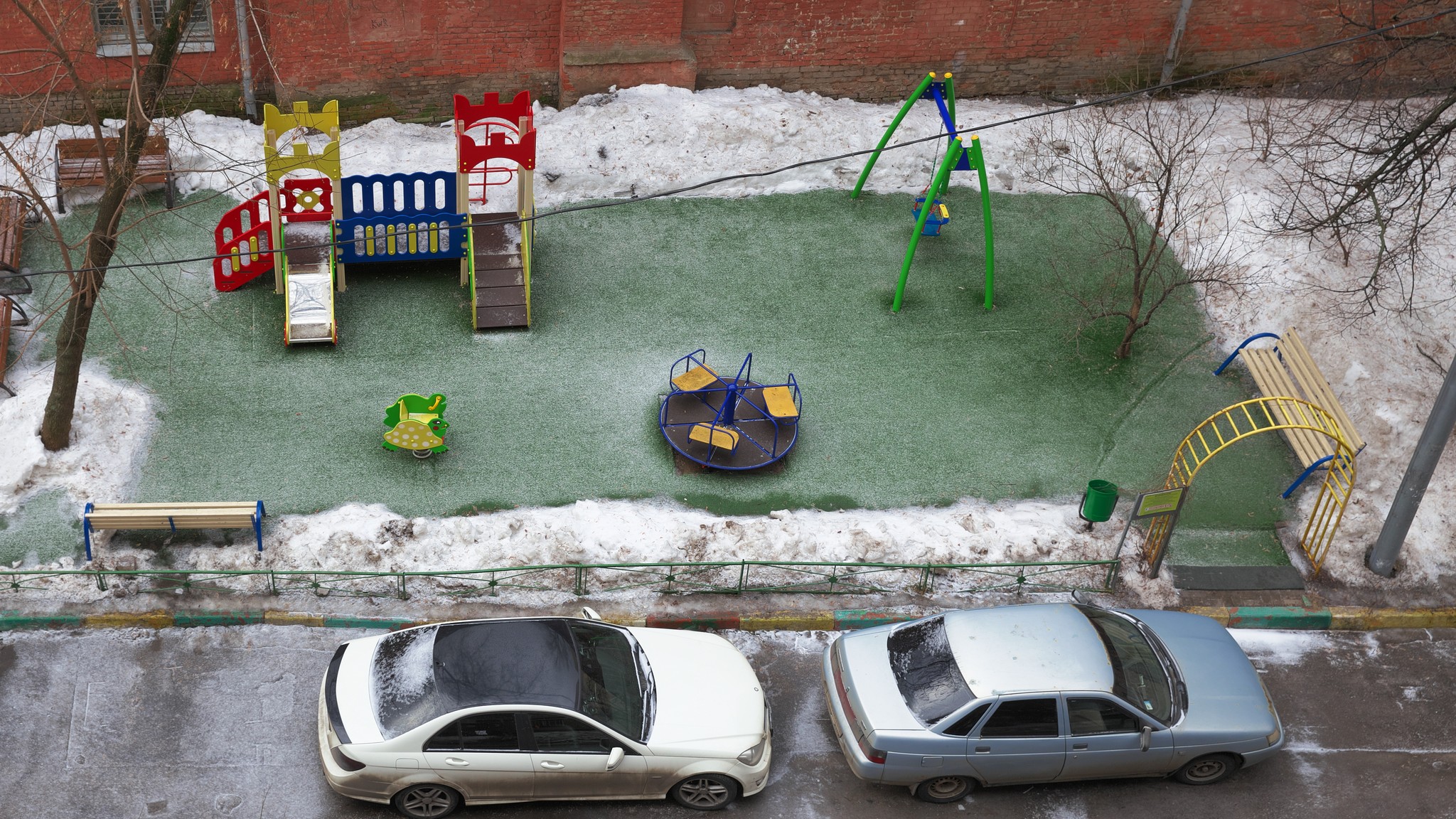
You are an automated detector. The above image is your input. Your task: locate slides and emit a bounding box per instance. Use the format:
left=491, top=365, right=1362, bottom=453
left=284, top=263, right=335, bottom=344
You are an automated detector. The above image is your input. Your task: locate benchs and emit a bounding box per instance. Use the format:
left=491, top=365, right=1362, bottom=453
left=54, top=135, right=176, bottom=214
left=1212, top=326, right=1366, bottom=499
left=0, top=185, right=42, bottom=397
left=84, top=500, right=266, bottom=561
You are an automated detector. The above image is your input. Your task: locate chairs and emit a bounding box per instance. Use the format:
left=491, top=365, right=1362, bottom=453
left=687, top=422, right=739, bottom=456
left=670, top=349, right=719, bottom=402
left=762, top=373, right=802, bottom=426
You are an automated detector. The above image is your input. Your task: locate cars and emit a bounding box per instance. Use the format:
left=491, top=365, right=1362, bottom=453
left=822, top=588, right=1284, bottom=804
left=317, top=606, right=775, bottom=819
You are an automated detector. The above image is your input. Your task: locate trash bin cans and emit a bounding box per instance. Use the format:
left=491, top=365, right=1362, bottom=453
left=1082, top=479, right=1118, bottom=523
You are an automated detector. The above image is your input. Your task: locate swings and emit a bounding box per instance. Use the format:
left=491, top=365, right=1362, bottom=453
left=911, top=120, right=953, bottom=237
